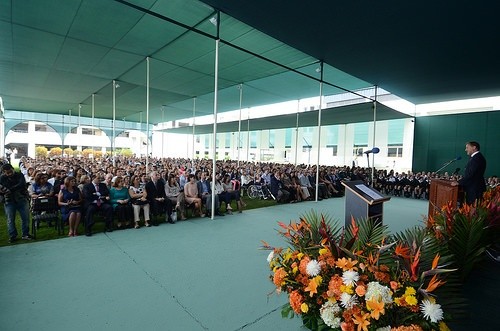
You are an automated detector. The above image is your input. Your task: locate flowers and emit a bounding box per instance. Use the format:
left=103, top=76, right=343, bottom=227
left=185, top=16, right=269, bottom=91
left=258, top=189, right=500, bottom=331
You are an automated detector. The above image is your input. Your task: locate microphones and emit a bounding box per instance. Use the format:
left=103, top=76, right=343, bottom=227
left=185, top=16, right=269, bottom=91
left=449, top=156, right=462, bottom=163
left=364, top=147, right=379, bottom=153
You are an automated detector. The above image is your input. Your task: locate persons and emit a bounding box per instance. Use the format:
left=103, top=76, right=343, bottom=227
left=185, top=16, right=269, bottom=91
left=0, top=156, right=500, bottom=243
left=451, top=141, right=487, bottom=207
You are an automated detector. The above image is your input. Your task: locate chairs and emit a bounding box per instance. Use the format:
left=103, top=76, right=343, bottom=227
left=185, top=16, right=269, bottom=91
left=32, top=195, right=60, bottom=237
